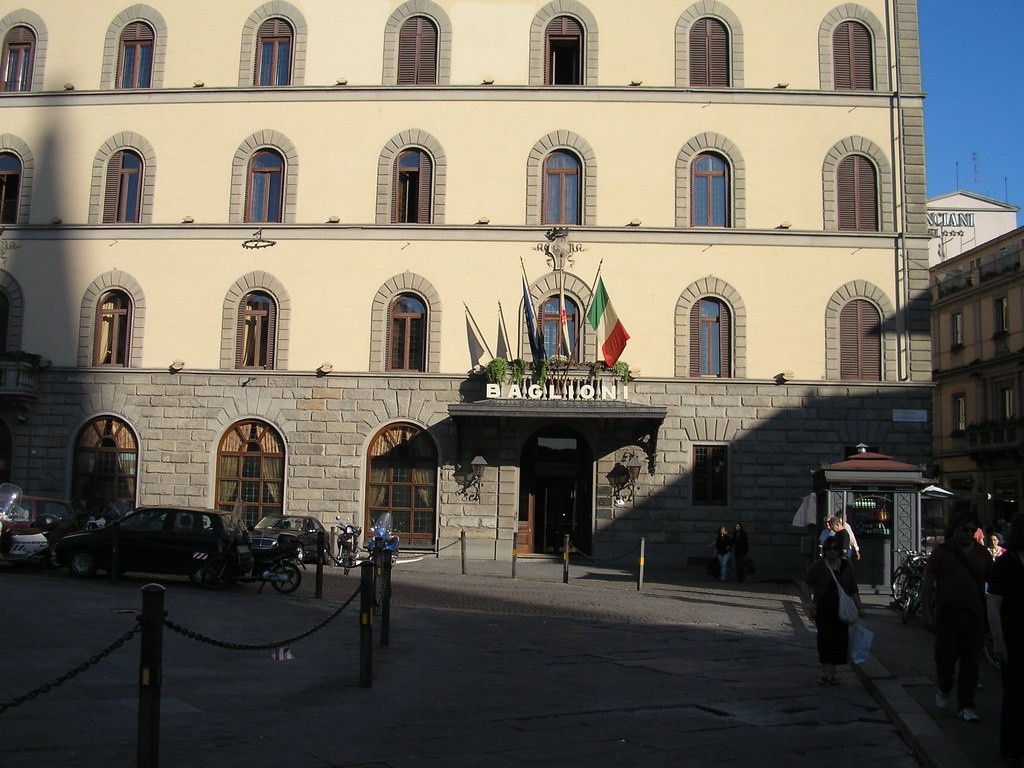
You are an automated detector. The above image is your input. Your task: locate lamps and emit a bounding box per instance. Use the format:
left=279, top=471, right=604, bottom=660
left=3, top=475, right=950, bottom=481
left=49, top=216, right=61, bottom=224
left=468, top=456, right=489, bottom=502
left=171, top=361, right=185, bottom=371
left=631, top=219, right=642, bottom=226
left=622, top=451, right=642, bottom=495
left=779, top=221, right=792, bottom=229
left=777, top=82, right=789, bottom=88
left=329, top=216, right=341, bottom=222
left=478, top=217, right=490, bottom=224
left=782, top=369, right=796, bottom=381
left=321, top=364, right=333, bottom=372
left=193, top=80, right=206, bottom=86
left=183, top=215, right=194, bottom=223
left=336, top=80, right=347, bottom=84
left=632, top=80, right=642, bottom=85
left=63, top=83, right=74, bottom=90
left=483, top=79, right=494, bottom=84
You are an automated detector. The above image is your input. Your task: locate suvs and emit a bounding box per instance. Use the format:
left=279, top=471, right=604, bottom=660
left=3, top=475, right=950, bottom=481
left=58, top=504, right=253, bottom=584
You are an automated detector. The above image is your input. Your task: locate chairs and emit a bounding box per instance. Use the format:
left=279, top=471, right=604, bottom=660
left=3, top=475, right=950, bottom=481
left=308, top=522, right=314, bottom=530
left=282, top=521, right=291, bottom=529
left=179, top=515, right=192, bottom=533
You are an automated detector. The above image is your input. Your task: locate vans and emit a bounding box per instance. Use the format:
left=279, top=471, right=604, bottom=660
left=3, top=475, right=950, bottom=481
left=0, top=495, right=79, bottom=543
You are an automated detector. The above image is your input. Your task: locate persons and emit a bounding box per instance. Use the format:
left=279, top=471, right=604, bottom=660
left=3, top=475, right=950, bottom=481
left=987, top=511, right=1024, bottom=768
left=918, top=506, right=1007, bottom=722
left=76, top=499, right=121, bottom=531
left=706, top=521, right=749, bottom=583
left=809, top=510, right=866, bottom=687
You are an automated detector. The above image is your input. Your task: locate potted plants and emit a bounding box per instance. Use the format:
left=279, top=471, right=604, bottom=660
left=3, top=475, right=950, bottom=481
left=485, top=356, right=633, bottom=387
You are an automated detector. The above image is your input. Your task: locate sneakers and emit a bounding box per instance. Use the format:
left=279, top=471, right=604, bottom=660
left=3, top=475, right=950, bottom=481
left=935, top=689, right=950, bottom=709
left=957, top=708, right=980, bottom=722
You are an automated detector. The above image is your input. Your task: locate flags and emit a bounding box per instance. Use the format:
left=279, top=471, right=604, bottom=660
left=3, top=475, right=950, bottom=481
left=521, top=273, right=544, bottom=378
left=587, top=275, right=631, bottom=368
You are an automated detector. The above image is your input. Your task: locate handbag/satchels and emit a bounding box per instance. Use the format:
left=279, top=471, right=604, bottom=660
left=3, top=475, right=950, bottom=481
left=705, top=557, right=721, bottom=576
left=847, top=619, right=875, bottom=667
left=839, top=593, right=858, bottom=625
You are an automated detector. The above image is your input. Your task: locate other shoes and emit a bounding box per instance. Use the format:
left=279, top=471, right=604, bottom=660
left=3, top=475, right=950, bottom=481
left=830, top=676, right=840, bottom=683
left=977, top=684, right=984, bottom=688
left=817, top=675, right=831, bottom=685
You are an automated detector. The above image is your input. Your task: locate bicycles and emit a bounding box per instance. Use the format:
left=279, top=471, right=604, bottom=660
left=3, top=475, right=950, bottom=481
left=890, top=541, right=934, bottom=624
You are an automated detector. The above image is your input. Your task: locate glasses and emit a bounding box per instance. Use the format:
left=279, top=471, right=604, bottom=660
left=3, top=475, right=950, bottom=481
left=825, top=546, right=838, bottom=551
left=961, top=527, right=977, bottom=534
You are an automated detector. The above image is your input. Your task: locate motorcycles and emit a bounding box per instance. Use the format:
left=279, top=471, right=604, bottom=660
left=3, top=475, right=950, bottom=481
left=197, top=496, right=307, bottom=595
left=366, top=511, right=400, bottom=568
left=333, top=515, right=363, bottom=566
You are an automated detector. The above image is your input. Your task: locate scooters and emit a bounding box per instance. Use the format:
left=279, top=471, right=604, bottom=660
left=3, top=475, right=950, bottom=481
left=0, top=482, right=61, bottom=571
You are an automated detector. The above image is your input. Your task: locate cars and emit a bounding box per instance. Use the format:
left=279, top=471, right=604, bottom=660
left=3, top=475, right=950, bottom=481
left=249, top=514, right=330, bottom=565
